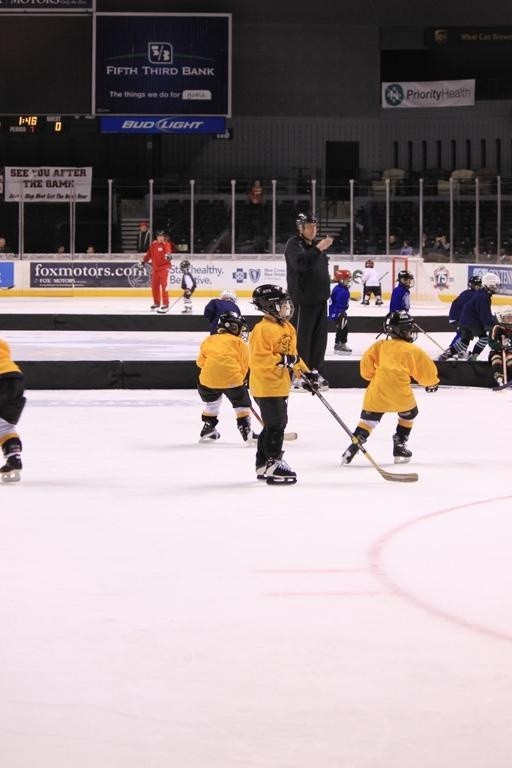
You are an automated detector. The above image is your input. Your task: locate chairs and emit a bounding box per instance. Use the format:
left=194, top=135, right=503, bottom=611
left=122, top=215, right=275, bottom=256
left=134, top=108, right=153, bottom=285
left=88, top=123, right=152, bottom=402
left=152, top=197, right=512, bottom=258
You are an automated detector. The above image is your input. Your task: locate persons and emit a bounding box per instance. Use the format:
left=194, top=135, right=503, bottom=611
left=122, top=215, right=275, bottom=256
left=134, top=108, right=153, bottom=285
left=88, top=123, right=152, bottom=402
left=0, top=337, right=27, bottom=473
left=439, top=275, right=482, bottom=361
left=344, top=309, right=440, bottom=465
left=143, top=230, right=174, bottom=313
left=86, top=241, right=96, bottom=253
left=136, top=223, right=152, bottom=253
left=362, top=260, right=382, bottom=304
left=441, top=272, right=501, bottom=364
left=179, top=260, right=196, bottom=315
left=248, top=179, right=264, bottom=206
left=390, top=231, right=512, bottom=264
left=247, top=284, right=319, bottom=479
left=0, top=237, right=13, bottom=253
left=56, top=244, right=65, bottom=253
left=284, top=211, right=333, bottom=389
left=195, top=310, right=261, bottom=443
left=204, top=290, right=241, bottom=335
left=390, top=270, right=416, bottom=313
left=328, top=267, right=353, bottom=352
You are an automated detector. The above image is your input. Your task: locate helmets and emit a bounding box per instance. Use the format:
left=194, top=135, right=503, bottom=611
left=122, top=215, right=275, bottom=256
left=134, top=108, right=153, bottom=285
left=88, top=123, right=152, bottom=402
left=495, top=305, right=512, bottom=325
left=468, top=272, right=501, bottom=294
left=222, top=290, right=235, bottom=302
left=396, top=270, right=414, bottom=289
left=383, top=309, right=418, bottom=342
left=181, top=261, right=190, bottom=272
left=252, top=284, right=295, bottom=324
left=296, top=212, right=319, bottom=224
left=366, top=260, right=374, bottom=268
left=335, top=269, right=352, bottom=287
left=216, top=310, right=249, bottom=342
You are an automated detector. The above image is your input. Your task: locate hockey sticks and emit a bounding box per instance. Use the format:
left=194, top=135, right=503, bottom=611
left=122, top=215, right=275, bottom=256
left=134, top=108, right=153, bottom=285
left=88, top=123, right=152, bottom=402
left=157, top=293, right=185, bottom=314
left=493, top=335, right=512, bottom=391
left=414, top=323, right=458, bottom=361
left=300, top=368, right=418, bottom=483
left=250, top=405, right=297, bottom=441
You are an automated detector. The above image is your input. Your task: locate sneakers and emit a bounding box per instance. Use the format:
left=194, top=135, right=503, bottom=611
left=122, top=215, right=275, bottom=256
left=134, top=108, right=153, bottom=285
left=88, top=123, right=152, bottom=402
left=363, top=298, right=383, bottom=304
left=257, top=458, right=297, bottom=478
left=151, top=304, right=168, bottom=310
left=182, top=309, right=191, bottom=313
left=292, top=370, right=328, bottom=388
left=334, top=342, right=352, bottom=352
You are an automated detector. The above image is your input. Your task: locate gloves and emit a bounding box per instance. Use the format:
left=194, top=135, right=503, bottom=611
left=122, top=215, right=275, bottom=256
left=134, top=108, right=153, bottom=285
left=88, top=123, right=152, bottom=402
left=281, top=354, right=300, bottom=368
left=302, top=371, right=319, bottom=395
left=500, top=334, right=511, bottom=349
left=426, top=384, right=438, bottom=393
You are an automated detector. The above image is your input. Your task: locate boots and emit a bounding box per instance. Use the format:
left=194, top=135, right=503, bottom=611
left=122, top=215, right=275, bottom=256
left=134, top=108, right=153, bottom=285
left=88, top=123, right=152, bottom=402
left=393, top=435, right=412, bottom=457
left=200, top=420, right=220, bottom=439
left=439, top=348, right=480, bottom=361
left=0, top=443, right=23, bottom=471
left=343, top=435, right=367, bottom=464
left=237, top=417, right=260, bottom=441
